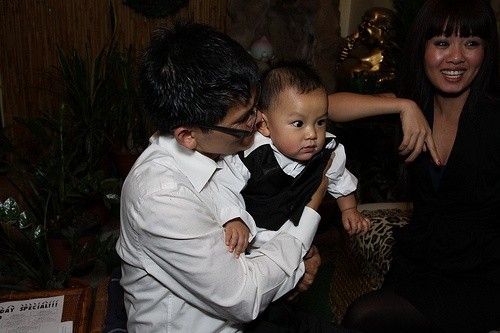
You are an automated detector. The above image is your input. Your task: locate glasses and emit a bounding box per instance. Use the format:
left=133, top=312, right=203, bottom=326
left=169, top=79, right=262, bottom=138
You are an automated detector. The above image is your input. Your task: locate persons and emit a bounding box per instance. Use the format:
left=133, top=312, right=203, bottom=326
left=117, top=22, right=331, bottom=333
left=209, top=63, right=372, bottom=325
left=327, top=2, right=500, bottom=333
